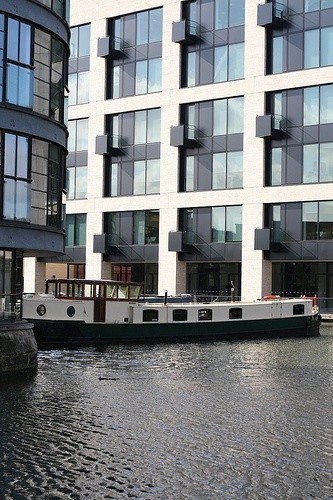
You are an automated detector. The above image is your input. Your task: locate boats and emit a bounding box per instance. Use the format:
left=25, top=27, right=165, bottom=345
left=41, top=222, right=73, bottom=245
left=22, top=275, right=323, bottom=349
